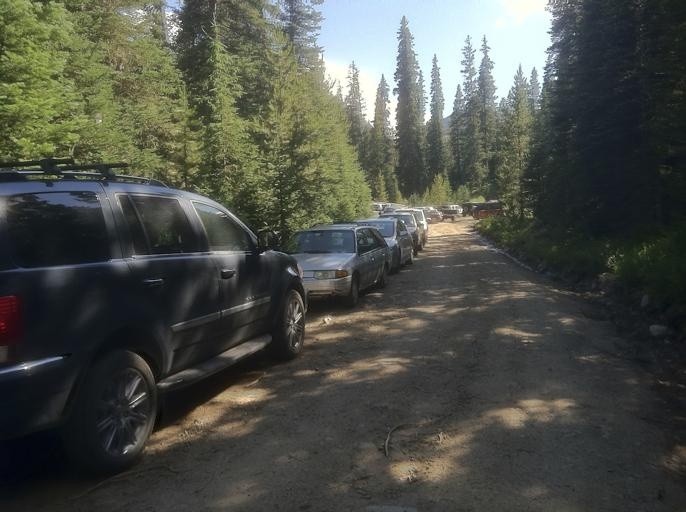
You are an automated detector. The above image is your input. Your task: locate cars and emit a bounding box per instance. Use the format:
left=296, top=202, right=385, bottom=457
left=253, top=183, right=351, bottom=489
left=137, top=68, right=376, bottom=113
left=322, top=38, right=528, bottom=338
left=282, top=223, right=388, bottom=307
left=356, top=198, right=463, bottom=273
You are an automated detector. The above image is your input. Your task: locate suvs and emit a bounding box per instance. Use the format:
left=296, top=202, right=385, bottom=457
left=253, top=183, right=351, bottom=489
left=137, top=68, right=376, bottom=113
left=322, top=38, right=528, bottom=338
left=0, top=179, right=306, bottom=478
